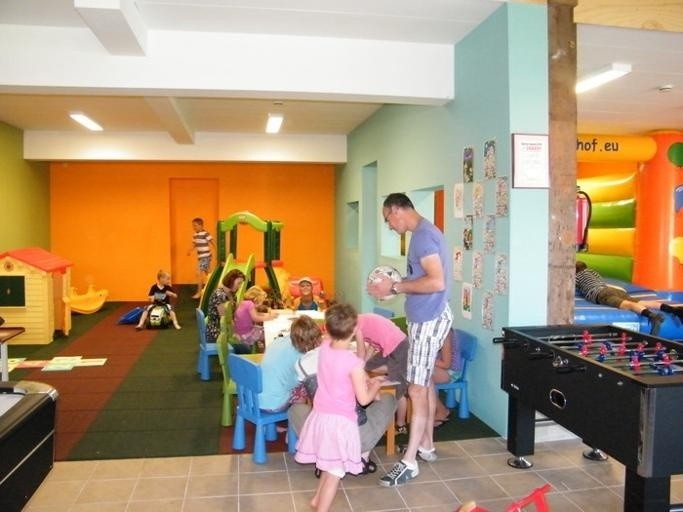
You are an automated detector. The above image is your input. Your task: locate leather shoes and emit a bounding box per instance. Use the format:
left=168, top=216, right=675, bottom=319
left=675, top=306, right=683, bottom=324
left=649, top=314, right=665, bottom=335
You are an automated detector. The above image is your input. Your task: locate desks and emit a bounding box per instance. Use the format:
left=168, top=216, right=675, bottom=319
left=305, top=371, right=401, bottom=456
left=264, top=309, right=326, bottom=352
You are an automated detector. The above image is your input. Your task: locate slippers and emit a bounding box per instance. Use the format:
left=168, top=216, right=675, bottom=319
left=432, top=418, right=453, bottom=430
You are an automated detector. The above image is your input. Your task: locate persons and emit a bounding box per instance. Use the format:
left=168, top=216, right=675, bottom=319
left=294, top=304, right=381, bottom=512
left=135, top=270, right=182, bottom=330
left=287, top=315, right=398, bottom=480
left=233, top=286, right=279, bottom=349
left=206, top=268, right=246, bottom=343
left=353, top=314, right=409, bottom=435
left=575, top=261, right=683, bottom=336
left=431, top=326, right=464, bottom=430
left=289, top=276, right=324, bottom=311
left=188, top=218, right=217, bottom=299
left=382, top=193, right=453, bottom=487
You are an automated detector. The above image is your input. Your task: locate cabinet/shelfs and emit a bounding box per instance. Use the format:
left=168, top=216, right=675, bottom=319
left=0, top=380, right=58, bottom=511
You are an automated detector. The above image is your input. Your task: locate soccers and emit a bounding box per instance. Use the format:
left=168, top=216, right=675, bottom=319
left=366, top=265, right=403, bottom=302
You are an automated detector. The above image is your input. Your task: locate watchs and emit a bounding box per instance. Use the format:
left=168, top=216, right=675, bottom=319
left=391, top=282, right=399, bottom=294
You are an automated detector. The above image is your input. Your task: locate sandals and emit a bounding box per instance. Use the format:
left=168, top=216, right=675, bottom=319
left=359, top=457, right=377, bottom=475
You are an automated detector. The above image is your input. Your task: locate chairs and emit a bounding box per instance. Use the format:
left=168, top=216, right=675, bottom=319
left=216, top=332, right=262, bottom=427
left=227, top=352, right=300, bottom=465
left=197, top=310, right=234, bottom=381
left=224, top=301, right=259, bottom=354
left=434, top=331, right=478, bottom=419
left=389, top=316, right=408, bottom=337
left=373, top=306, right=394, bottom=319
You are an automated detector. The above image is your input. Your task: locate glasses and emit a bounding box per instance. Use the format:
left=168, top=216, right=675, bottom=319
left=385, top=210, right=393, bottom=222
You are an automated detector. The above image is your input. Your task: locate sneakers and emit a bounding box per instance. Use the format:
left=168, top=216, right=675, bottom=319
left=403, top=446, right=438, bottom=463
left=393, top=424, right=408, bottom=436
left=379, top=460, right=419, bottom=487
